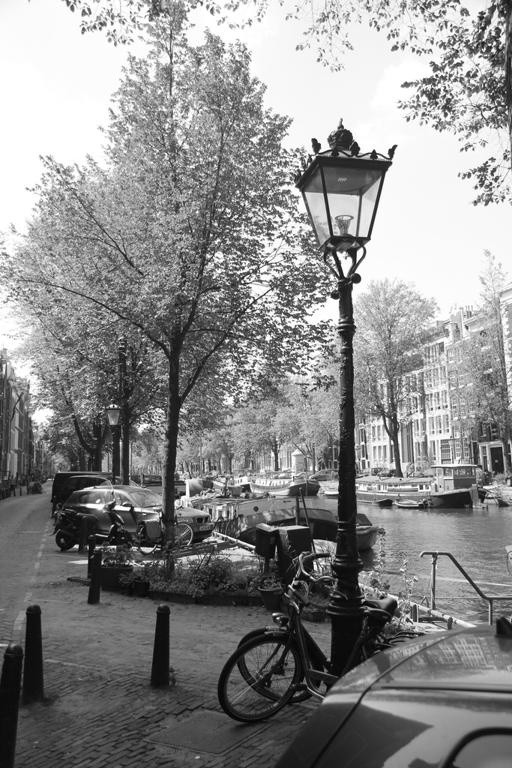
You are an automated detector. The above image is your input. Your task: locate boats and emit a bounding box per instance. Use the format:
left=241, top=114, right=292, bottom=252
left=325, top=463, right=481, bottom=508
left=249, top=476, right=320, bottom=496
left=375, top=497, right=393, bottom=506
left=212, top=473, right=248, bottom=497
left=394, top=497, right=429, bottom=508
left=172, top=489, right=379, bottom=550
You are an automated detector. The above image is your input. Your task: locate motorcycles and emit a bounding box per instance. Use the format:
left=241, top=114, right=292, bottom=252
left=52, top=490, right=132, bottom=550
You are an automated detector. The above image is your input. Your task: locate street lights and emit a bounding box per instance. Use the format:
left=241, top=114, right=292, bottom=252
left=104, top=402, right=123, bottom=484
left=290, top=117, right=396, bottom=680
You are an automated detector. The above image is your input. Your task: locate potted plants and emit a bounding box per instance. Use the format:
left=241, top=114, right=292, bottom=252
left=101, top=542, right=150, bottom=596
left=258, top=581, right=286, bottom=612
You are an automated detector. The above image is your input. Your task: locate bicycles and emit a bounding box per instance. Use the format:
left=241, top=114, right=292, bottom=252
left=218, top=551, right=427, bottom=724
left=137, top=502, right=195, bottom=556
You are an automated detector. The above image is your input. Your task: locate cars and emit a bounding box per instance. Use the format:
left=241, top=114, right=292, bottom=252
left=277, top=618, right=512, bottom=768
left=64, top=484, right=214, bottom=547
left=51, top=469, right=140, bottom=509
